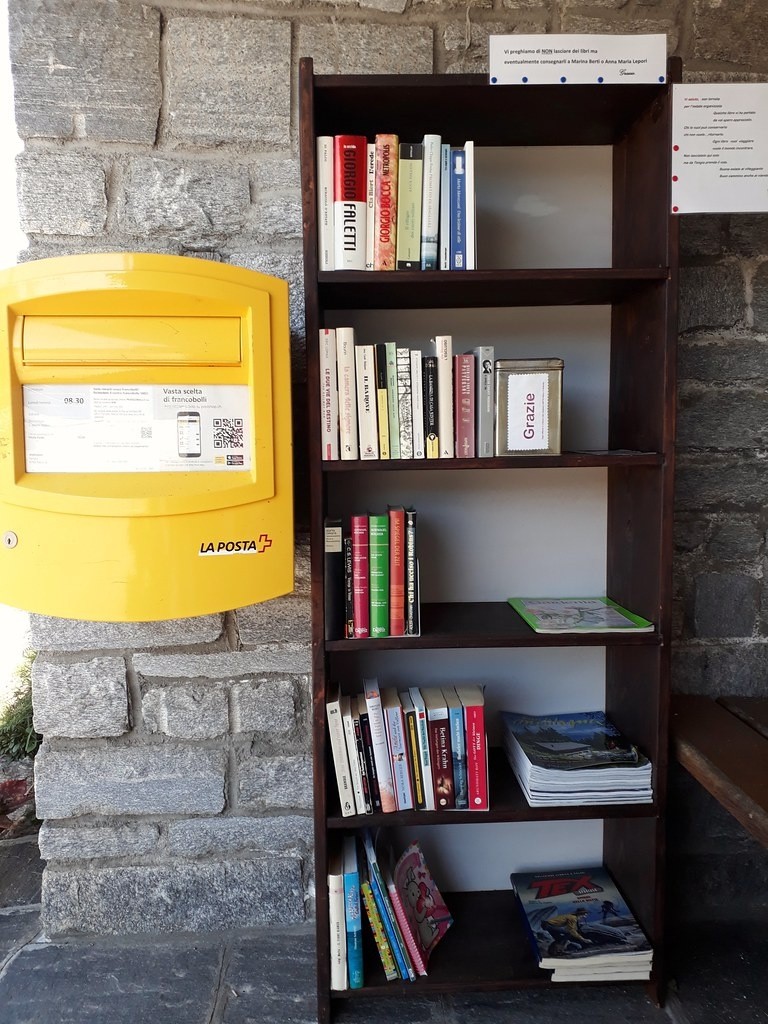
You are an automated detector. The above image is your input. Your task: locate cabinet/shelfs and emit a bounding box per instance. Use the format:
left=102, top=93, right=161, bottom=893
left=297, top=56, right=676, bottom=1024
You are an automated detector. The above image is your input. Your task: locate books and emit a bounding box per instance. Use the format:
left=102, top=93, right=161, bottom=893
left=316, top=133, right=656, bottom=991
